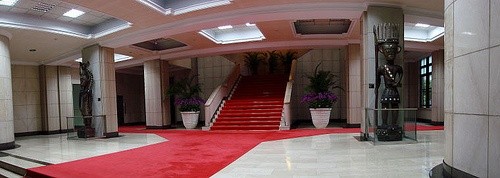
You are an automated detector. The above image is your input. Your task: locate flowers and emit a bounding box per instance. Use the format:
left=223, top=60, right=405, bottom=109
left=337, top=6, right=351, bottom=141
left=165, top=73, right=206, bottom=113
left=299, top=62, right=345, bottom=109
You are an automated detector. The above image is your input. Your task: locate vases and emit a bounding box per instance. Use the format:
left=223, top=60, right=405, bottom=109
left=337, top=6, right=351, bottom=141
left=180, top=110, right=201, bottom=129
left=309, top=108, right=332, bottom=129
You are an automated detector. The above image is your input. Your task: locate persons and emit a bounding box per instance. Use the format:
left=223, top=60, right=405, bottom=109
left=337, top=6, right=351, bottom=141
left=77, top=62, right=93, bottom=127
left=376, top=42, right=403, bottom=128
